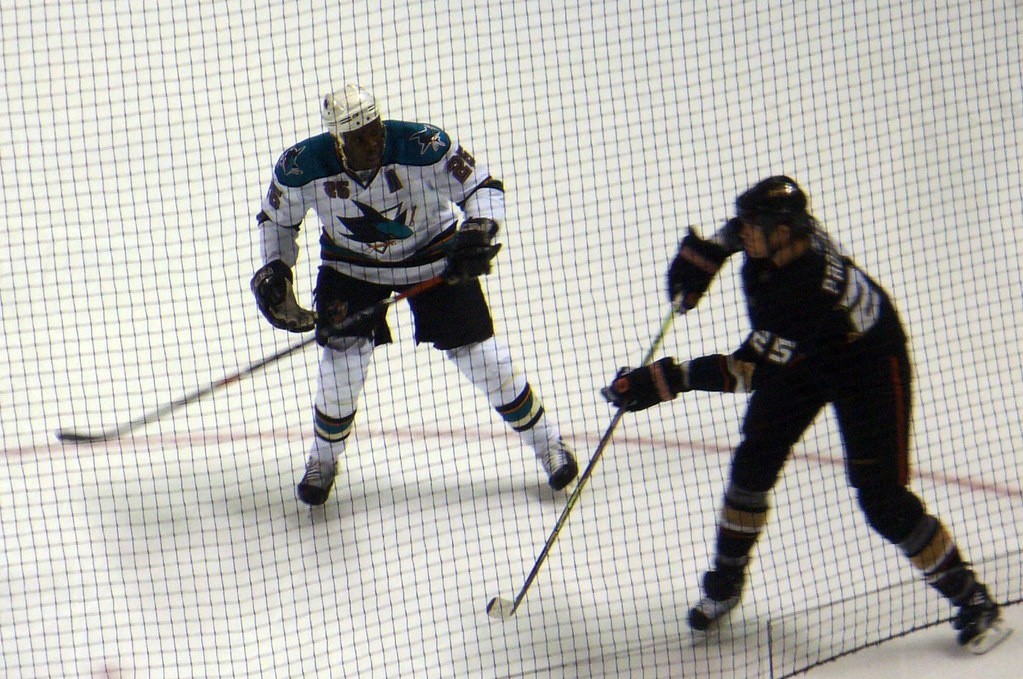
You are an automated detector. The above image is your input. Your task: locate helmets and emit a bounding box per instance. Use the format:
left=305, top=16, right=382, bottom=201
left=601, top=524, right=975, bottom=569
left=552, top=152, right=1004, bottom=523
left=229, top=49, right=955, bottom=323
left=322, top=82, right=383, bottom=144
left=735, top=175, right=805, bottom=236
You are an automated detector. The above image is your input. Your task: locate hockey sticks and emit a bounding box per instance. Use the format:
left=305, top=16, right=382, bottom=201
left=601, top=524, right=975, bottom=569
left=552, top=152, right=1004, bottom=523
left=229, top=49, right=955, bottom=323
left=55, top=242, right=502, bottom=449
left=485, top=292, right=684, bottom=620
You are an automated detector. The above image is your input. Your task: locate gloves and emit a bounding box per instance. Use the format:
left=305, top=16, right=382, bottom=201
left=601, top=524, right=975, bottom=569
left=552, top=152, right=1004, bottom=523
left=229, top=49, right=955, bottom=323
left=666, top=223, right=730, bottom=313
left=249, top=259, right=319, bottom=332
left=600, top=357, right=681, bottom=413
left=439, top=217, right=499, bottom=284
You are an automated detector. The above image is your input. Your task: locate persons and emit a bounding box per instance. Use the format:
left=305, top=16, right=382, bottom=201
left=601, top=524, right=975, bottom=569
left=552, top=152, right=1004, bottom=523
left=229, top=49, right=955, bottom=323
left=600, top=176, right=1002, bottom=647
left=251, top=83, right=578, bottom=505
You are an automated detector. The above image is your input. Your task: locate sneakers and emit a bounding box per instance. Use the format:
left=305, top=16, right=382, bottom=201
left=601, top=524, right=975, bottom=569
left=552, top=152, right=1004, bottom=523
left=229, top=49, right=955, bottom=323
left=687, top=592, right=739, bottom=644
left=298, top=456, right=336, bottom=516
left=952, top=583, right=1014, bottom=655
left=541, top=436, right=579, bottom=495
